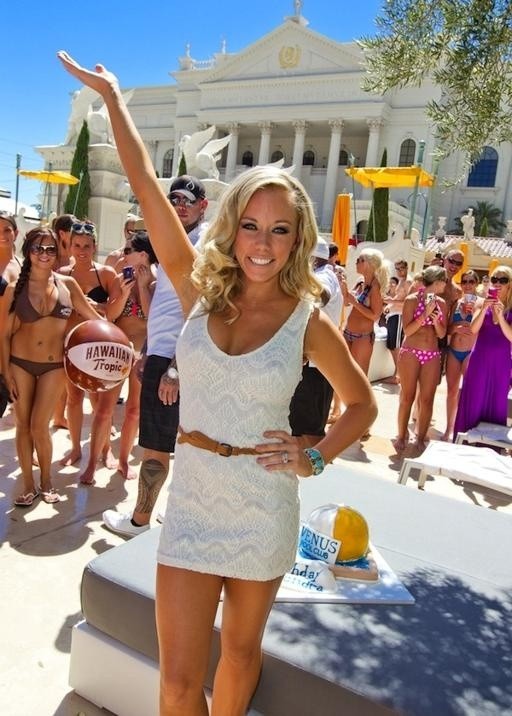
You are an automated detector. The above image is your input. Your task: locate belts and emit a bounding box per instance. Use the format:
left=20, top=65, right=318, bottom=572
left=176, top=425, right=282, bottom=456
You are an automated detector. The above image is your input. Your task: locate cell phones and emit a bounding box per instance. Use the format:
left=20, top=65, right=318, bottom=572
left=488, top=288, right=498, bottom=303
left=426, top=292, right=434, bottom=303
left=123, top=266, right=134, bottom=285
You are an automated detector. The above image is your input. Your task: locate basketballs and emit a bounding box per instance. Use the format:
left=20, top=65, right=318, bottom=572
left=63, top=319, right=135, bottom=394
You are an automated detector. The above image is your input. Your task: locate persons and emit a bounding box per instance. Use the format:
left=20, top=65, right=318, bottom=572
left=288, top=236, right=512, bottom=451
left=58, top=49, right=378, bottom=715
left=0, top=177, right=212, bottom=534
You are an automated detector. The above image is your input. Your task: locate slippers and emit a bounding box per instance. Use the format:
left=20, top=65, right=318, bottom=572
left=37, top=482, right=60, bottom=503
left=14, top=490, right=39, bottom=506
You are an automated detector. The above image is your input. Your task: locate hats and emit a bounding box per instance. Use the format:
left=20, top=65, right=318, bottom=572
left=312, top=236, right=330, bottom=260
left=126, top=220, right=148, bottom=240
left=166, top=174, right=205, bottom=201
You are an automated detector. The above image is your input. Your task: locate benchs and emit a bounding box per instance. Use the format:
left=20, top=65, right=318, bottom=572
left=398, top=440, right=511, bottom=510
left=455, top=422, right=511, bottom=457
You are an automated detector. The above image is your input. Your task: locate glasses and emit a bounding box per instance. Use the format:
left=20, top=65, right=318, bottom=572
left=71, top=221, right=95, bottom=235
left=29, top=245, right=58, bottom=256
left=490, top=277, right=509, bottom=285
left=447, top=257, right=462, bottom=266
left=462, top=280, right=475, bottom=285
left=170, top=195, right=198, bottom=208
left=395, top=266, right=405, bottom=270
left=124, top=247, right=136, bottom=255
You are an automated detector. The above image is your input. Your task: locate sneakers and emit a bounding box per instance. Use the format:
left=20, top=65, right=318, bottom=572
left=102, top=509, right=150, bottom=538
left=158, top=509, right=166, bottom=523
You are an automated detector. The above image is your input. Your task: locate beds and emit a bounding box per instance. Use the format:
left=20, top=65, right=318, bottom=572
left=68, top=460, right=512, bottom=716
